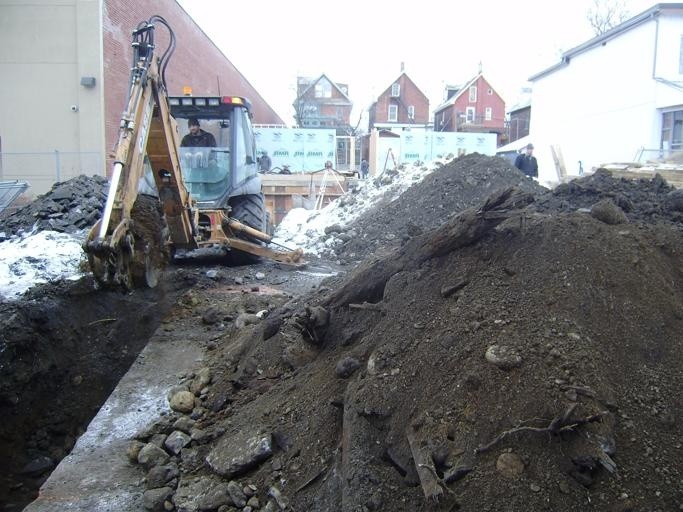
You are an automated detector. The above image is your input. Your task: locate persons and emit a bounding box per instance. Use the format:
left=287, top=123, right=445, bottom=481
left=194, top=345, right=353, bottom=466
left=361, top=158, right=369, bottom=180
left=177, top=117, right=219, bottom=199
left=258, top=150, right=272, bottom=171
left=514, top=143, right=539, bottom=179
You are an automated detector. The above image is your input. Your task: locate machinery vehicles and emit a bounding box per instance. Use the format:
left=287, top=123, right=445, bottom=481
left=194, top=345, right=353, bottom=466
left=79, top=13, right=310, bottom=290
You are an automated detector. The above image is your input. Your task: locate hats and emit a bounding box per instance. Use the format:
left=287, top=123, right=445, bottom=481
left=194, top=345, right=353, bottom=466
left=188, top=118, right=200, bottom=126
